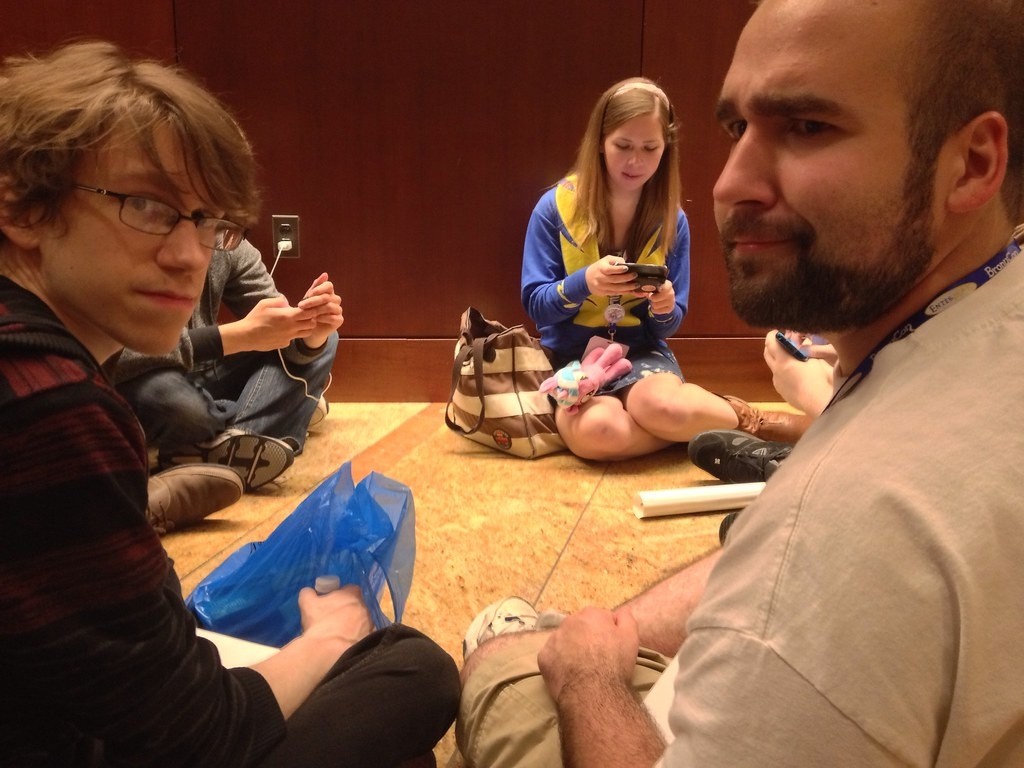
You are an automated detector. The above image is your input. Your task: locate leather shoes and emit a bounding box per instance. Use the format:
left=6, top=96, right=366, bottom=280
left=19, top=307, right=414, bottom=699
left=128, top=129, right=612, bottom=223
left=145, top=463, right=245, bottom=536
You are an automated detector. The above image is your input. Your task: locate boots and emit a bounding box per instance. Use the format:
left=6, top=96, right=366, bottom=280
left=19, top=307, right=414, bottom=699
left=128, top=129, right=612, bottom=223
left=724, top=394, right=814, bottom=443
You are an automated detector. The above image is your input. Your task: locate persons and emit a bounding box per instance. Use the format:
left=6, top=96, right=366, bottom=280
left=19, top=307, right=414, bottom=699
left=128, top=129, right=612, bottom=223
left=100, top=228, right=343, bottom=537
left=0, top=42, right=460, bottom=768
left=689, top=321, right=840, bottom=485
left=521, top=75, right=813, bottom=464
left=455, top=1, right=1023, bottom=767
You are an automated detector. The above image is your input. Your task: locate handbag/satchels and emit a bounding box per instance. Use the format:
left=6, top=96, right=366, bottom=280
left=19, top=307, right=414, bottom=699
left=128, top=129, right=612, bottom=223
left=445, top=306, right=568, bottom=461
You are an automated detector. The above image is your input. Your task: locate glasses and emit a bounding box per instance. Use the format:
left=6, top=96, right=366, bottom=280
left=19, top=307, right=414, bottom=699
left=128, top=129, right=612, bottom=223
left=61, top=179, right=249, bottom=252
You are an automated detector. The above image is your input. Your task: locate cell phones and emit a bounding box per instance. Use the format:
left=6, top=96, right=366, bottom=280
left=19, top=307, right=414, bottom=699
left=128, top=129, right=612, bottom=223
left=775, top=332, right=808, bottom=361
left=614, top=263, right=669, bottom=292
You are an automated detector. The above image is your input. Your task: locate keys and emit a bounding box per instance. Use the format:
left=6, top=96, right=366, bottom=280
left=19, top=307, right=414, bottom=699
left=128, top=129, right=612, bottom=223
left=608, top=328, right=616, bottom=341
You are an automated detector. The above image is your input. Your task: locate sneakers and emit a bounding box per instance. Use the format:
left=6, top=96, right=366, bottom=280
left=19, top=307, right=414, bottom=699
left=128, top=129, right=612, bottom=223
left=688, top=428, right=793, bottom=484
left=157, top=428, right=295, bottom=490
left=462, top=594, right=540, bottom=664
left=719, top=512, right=740, bottom=546
left=307, top=395, right=329, bottom=428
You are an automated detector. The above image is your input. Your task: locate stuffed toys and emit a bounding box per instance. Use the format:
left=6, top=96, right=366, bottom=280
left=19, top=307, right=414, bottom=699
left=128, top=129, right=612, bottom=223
left=539, top=344, right=632, bottom=416
